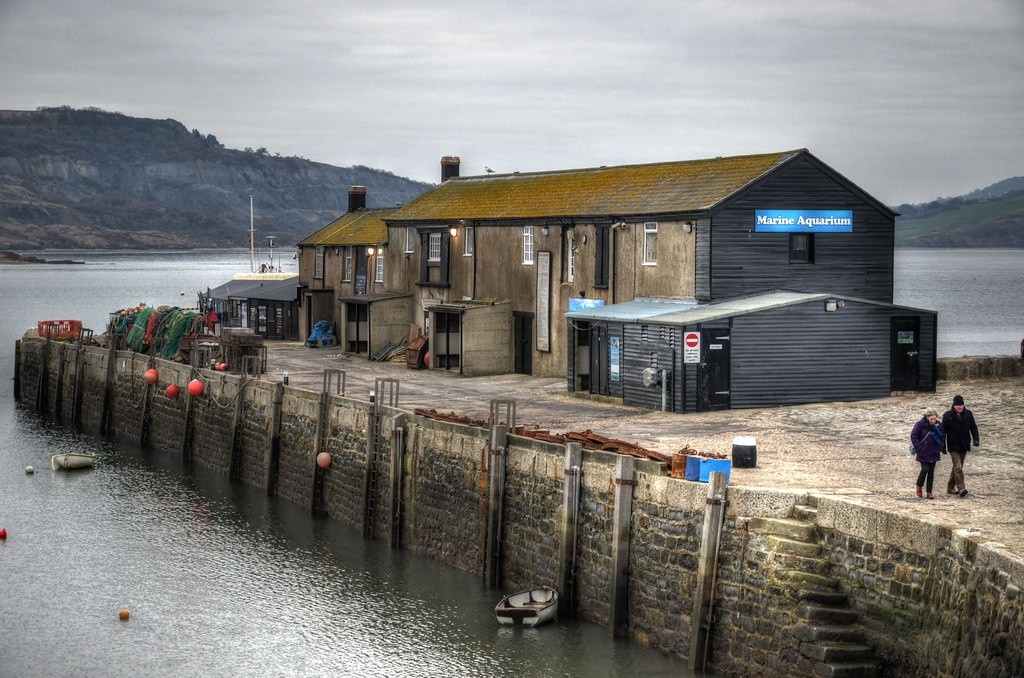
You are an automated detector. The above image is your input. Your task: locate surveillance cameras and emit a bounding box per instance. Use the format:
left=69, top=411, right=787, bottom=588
left=622, top=222, right=627, bottom=228
left=612, top=222, right=622, bottom=229
left=293, top=255, right=296, bottom=259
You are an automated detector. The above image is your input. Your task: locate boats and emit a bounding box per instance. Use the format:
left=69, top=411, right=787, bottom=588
left=493, top=583, right=559, bottom=629
left=50, top=453, right=98, bottom=473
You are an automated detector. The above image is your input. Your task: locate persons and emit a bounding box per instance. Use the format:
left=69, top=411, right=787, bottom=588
left=911, top=408, right=942, bottom=499
left=941, top=395, right=979, bottom=497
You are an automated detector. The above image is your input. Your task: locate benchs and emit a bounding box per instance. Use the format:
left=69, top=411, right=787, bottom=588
left=685, top=454, right=732, bottom=485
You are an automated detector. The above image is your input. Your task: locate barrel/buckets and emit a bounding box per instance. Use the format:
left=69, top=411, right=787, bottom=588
left=671, top=454, right=689, bottom=479
left=685, top=456, right=704, bottom=481
left=732, top=444, right=756, bottom=468
left=699, top=458, right=731, bottom=484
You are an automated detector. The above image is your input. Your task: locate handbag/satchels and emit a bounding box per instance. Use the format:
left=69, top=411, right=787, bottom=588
left=908, top=442, right=917, bottom=455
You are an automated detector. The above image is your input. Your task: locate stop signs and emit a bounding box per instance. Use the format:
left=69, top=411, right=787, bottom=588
left=686, top=334, right=699, bottom=347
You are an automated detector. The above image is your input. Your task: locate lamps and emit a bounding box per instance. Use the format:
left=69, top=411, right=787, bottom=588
left=450, top=225, right=458, bottom=236
left=826, top=299, right=837, bottom=312
left=683, top=221, right=692, bottom=233
left=368, top=246, right=374, bottom=256
left=541, top=224, right=549, bottom=236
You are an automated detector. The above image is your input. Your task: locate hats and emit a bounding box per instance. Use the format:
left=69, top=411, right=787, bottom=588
left=953, top=395, right=964, bottom=405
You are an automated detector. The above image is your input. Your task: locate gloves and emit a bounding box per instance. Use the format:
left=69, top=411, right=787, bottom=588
left=973, top=440, right=979, bottom=446
left=942, top=449, right=947, bottom=454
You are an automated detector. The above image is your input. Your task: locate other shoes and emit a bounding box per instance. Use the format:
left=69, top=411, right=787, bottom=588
left=927, top=492, right=934, bottom=499
left=916, top=483, right=922, bottom=497
left=948, top=488, right=959, bottom=494
left=959, top=489, right=968, bottom=496
